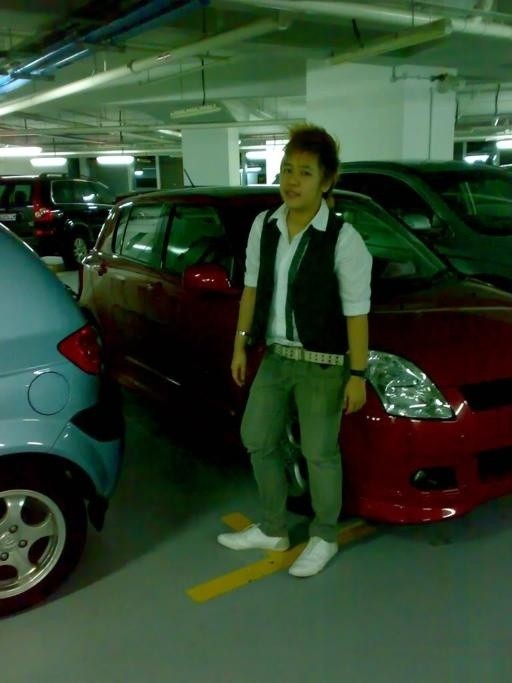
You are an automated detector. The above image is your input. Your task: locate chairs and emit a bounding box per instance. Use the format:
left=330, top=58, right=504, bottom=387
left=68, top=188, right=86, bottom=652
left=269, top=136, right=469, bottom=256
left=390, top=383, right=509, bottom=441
left=13, top=192, right=28, bottom=201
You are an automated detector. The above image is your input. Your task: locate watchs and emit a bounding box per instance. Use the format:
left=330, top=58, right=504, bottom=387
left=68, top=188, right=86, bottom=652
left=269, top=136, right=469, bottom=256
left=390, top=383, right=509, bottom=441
left=348, top=366, right=370, bottom=379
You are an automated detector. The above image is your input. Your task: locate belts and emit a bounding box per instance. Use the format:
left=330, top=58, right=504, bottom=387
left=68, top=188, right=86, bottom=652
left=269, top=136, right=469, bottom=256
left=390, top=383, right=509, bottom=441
left=273, top=343, right=345, bottom=366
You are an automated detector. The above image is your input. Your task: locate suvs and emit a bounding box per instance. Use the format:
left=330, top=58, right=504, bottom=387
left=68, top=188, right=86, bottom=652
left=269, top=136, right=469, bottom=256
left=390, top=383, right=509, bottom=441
left=0, top=172, right=116, bottom=269
left=334, top=161, right=511, bottom=285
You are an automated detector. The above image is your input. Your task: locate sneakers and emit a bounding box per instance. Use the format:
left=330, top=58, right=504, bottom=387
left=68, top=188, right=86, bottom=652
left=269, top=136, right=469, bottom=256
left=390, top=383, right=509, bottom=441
left=216, top=522, right=290, bottom=552
left=288, top=536, right=339, bottom=577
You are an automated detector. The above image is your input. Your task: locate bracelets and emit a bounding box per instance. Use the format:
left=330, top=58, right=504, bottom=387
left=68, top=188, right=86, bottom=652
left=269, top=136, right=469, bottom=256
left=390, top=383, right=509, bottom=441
left=234, top=328, right=253, bottom=338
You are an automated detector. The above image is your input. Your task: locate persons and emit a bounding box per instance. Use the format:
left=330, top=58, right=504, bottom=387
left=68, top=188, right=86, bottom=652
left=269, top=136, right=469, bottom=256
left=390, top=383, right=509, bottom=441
left=213, top=117, right=375, bottom=581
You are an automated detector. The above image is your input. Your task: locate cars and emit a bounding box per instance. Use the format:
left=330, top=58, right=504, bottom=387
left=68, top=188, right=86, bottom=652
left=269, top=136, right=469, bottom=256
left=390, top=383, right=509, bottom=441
left=83, top=184, right=511, bottom=527
left=0, top=223, right=124, bottom=616
